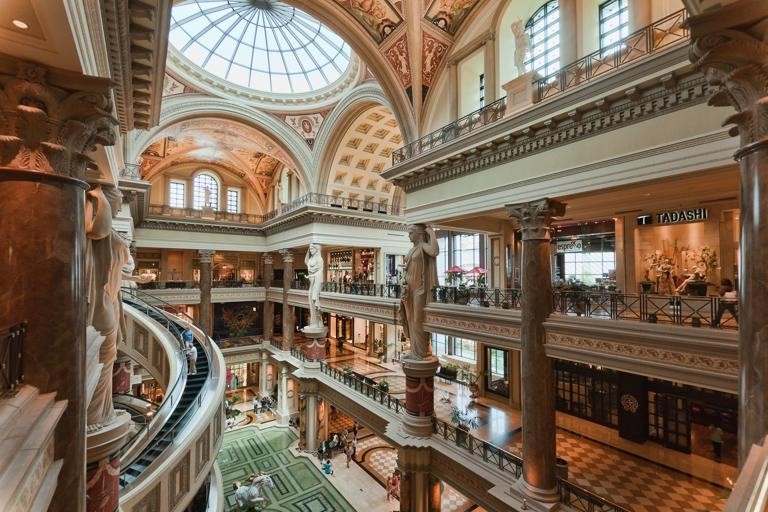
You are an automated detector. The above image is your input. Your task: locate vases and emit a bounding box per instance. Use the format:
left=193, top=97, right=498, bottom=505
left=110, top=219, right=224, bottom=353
left=691, top=316, right=702, bottom=327
left=648, top=314, right=658, bottom=323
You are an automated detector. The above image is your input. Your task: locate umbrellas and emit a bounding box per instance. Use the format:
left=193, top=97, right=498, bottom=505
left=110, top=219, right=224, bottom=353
left=444, top=266, right=465, bottom=273
left=466, top=267, right=487, bottom=273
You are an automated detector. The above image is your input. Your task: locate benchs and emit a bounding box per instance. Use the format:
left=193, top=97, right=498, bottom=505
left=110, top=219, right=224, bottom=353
left=265, top=396, right=278, bottom=415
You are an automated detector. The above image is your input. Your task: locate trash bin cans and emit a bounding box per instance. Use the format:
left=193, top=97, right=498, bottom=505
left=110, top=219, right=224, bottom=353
left=556, top=458, right=569, bottom=480
left=380, top=382, right=388, bottom=392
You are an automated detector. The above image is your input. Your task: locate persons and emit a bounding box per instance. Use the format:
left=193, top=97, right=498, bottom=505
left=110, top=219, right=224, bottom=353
left=252, top=397, right=274, bottom=414
left=318, top=422, right=358, bottom=474
left=289, top=416, right=300, bottom=428
left=336, top=336, right=343, bottom=352
left=304, top=244, right=324, bottom=328
left=389, top=269, right=399, bottom=298
left=326, top=339, right=333, bottom=356
left=343, top=270, right=368, bottom=292
left=709, top=420, right=724, bottom=460
left=712, top=279, right=739, bottom=327
left=659, top=259, right=674, bottom=294
left=374, top=338, right=384, bottom=351
left=85, top=185, right=153, bottom=433
left=675, top=266, right=703, bottom=293
left=183, top=343, right=197, bottom=375
left=398, top=224, right=439, bottom=361
left=118, top=232, right=138, bottom=289
left=386, top=472, right=402, bottom=501
left=180, top=327, right=193, bottom=355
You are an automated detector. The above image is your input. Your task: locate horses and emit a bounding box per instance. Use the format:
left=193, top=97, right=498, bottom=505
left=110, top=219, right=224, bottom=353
left=235, top=474, right=274, bottom=509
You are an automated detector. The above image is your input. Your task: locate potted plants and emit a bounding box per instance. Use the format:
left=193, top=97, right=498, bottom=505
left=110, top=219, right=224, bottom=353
left=451, top=405, right=488, bottom=443
left=568, top=295, right=591, bottom=316
